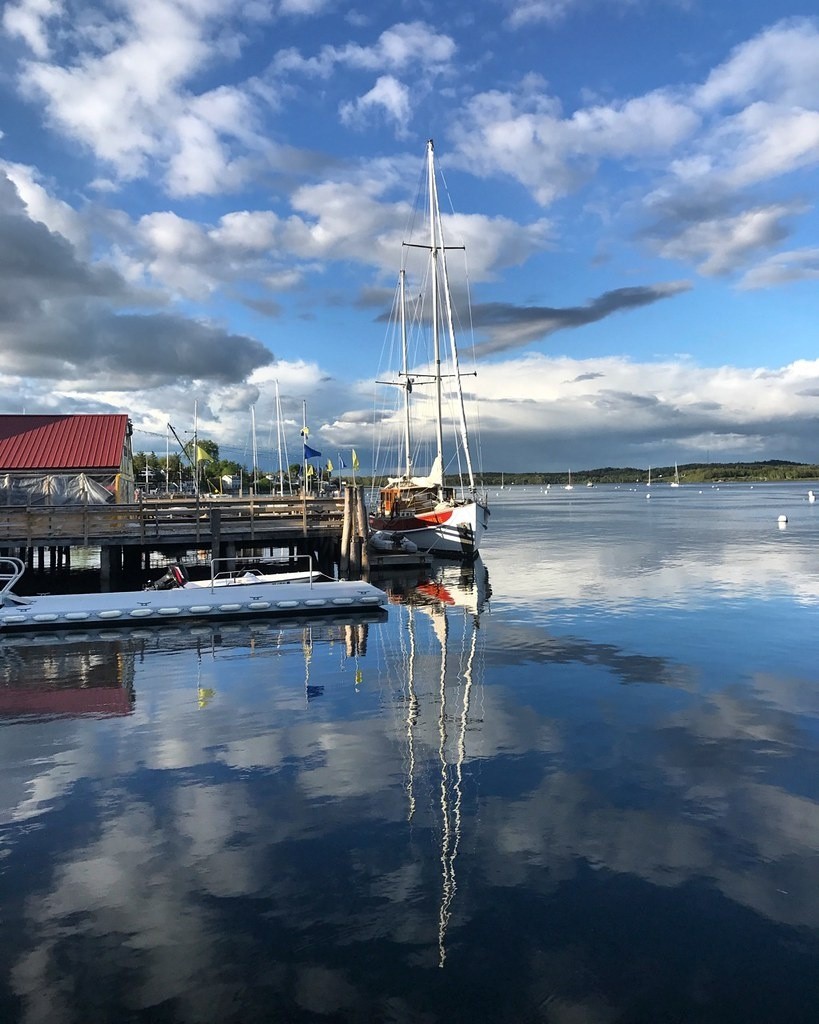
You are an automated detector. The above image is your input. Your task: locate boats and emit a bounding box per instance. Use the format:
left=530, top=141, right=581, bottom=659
left=173, top=569, right=323, bottom=587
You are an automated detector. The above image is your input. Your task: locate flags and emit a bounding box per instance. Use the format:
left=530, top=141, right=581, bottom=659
left=307, top=465, right=314, bottom=477
left=352, top=450, right=360, bottom=471
left=301, top=427, right=309, bottom=440
left=303, top=443, right=321, bottom=459
left=306, top=685, right=324, bottom=703
left=339, top=456, right=346, bottom=469
left=194, top=446, right=214, bottom=463
left=199, top=689, right=216, bottom=709
left=297, top=465, right=303, bottom=481
left=355, top=672, right=364, bottom=685
left=326, top=458, right=333, bottom=471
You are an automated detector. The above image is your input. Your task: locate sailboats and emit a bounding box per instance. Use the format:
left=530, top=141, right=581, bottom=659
left=383, top=561, right=492, bottom=970
left=364, top=138, right=491, bottom=561
